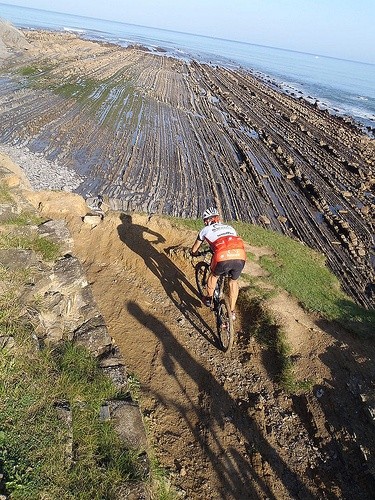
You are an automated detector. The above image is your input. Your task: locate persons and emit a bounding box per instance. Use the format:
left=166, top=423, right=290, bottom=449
left=191, top=207, right=246, bottom=321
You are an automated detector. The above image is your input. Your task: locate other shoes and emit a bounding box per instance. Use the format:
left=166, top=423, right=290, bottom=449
left=225, top=311, right=237, bottom=321
left=201, top=296, right=211, bottom=307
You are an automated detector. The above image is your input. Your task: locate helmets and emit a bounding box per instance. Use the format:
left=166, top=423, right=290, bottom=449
left=203, top=206, right=219, bottom=220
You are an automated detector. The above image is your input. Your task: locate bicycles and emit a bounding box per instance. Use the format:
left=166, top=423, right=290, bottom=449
left=190, top=247, right=234, bottom=356
